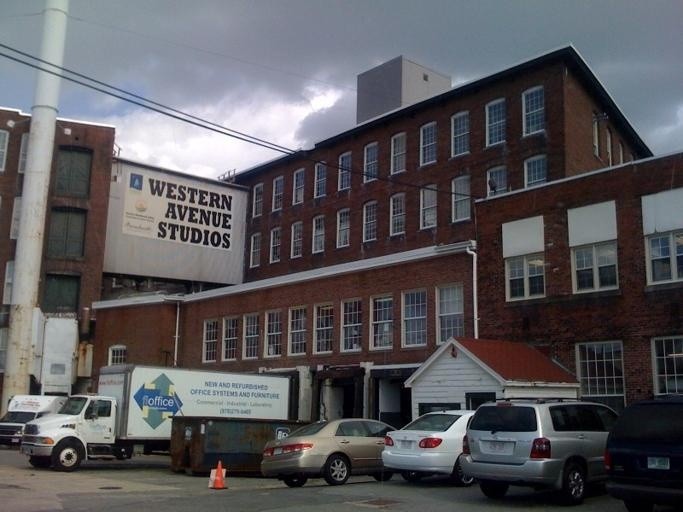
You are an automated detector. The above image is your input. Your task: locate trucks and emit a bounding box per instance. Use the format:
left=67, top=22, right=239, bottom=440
left=19, top=363, right=292, bottom=472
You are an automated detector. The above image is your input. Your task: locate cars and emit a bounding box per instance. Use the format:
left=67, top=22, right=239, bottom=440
left=378, top=408, right=476, bottom=488
left=261, top=416, right=399, bottom=486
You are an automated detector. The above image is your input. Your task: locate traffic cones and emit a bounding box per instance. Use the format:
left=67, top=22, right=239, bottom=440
left=211, top=460, right=228, bottom=490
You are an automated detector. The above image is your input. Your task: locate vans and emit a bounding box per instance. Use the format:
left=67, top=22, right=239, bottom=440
left=458, top=397, right=625, bottom=506
left=602, top=393, right=683, bottom=512
left=0, top=394, right=69, bottom=447
